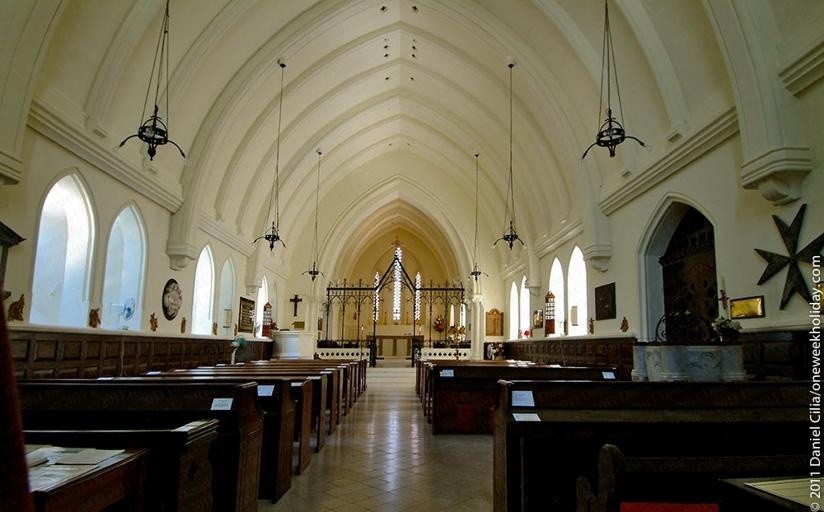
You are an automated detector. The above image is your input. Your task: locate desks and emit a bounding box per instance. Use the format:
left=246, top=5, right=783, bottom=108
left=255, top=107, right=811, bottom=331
left=0, top=331, right=824, bottom=512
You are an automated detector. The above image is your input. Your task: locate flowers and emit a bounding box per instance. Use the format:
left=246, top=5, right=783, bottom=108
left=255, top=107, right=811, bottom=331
left=432, top=313, right=447, bottom=340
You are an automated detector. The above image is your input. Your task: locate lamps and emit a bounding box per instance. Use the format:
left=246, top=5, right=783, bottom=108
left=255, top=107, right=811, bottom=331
left=467, top=152, right=490, bottom=283
left=113, top=0, right=186, bottom=161
left=491, top=60, right=527, bottom=251
left=251, top=57, right=288, bottom=252
left=302, top=148, right=327, bottom=283
left=578, top=0, right=652, bottom=161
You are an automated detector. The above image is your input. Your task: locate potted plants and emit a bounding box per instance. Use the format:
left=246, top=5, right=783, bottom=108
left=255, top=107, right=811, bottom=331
left=630, top=308, right=747, bottom=383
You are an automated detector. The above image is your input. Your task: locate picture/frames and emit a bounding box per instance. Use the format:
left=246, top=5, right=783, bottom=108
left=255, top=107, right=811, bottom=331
left=238, top=296, right=255, bottom=333
left=595, top=282, right=616, bottom=320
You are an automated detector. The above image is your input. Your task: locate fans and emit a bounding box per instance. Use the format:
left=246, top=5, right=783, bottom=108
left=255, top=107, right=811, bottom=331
left=109, top=298, right=135, bottom=330
